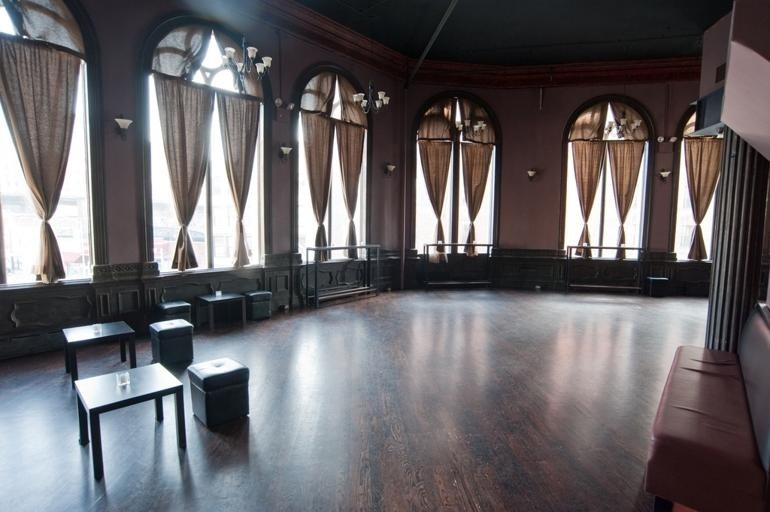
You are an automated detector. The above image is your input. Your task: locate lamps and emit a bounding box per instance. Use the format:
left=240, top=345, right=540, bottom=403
left=655, top=136, right=677, bottom=153
left=114, top=113, right=133, bottom=141
left=526, top=167, right=536, bottom=181
left=278, top=142, right=293, bottom=162
left=353, top=77, right=391, bottom=115
left=454, top=97, right=487, bottom=136
left=603, top=74, right=643, bottom=141
left=385, top=161, right=396, bottom=177
left=660, top=169, right=671, bottom=182
left=221, top=33, right=273, bottom=99
left=274, top=98, right=294, bottom=126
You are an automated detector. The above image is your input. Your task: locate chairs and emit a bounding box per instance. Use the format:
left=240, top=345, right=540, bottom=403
left=646, top=251, right=674, bottom=297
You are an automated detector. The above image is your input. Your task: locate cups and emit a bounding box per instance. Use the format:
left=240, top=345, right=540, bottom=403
left=117, top=372, right=130, bottom=387
left=437, top=240, right=443, bottom=245
left=94, top=325, right=101, bottom=334
left=621, top=243, right=626, bottom=248
left=216, top=291, right=223, bottom=298
left=583, top=243, right=588, bottom=246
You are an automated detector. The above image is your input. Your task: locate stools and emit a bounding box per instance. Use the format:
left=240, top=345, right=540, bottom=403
left=156, top=300, right=192, bottom=324
left=188, top=358, right=250, bottom=428
left=241, top=289, right=272, bottom=321
left=149, top=319, right=193, bottom=366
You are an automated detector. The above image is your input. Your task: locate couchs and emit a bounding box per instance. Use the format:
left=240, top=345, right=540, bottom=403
left=646, top=298, right=769, bottom=512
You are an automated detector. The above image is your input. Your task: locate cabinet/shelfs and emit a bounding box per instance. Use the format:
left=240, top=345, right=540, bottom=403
left=306, top=244, right=381, bottom=309
left=423, top=244, right=493, bottom=289
left=566, top=246, right=646, bottom=295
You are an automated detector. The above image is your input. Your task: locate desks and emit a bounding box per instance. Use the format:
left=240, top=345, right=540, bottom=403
left=194, top=292, right=246, bottom=332
left=488, top=248, right=566, bottom=290
left=74, top=362, right=186, bottom=482
left=62, top=320, right=136, bottom=389
